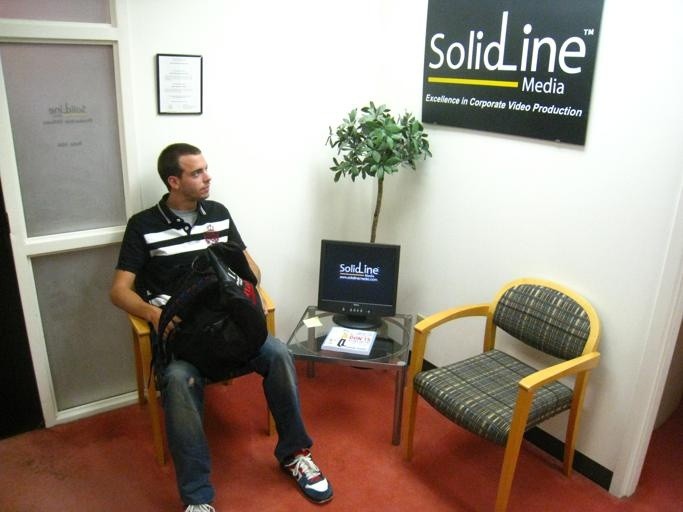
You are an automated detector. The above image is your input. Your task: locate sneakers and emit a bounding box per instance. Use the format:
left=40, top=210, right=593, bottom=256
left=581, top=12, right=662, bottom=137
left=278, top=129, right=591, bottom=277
left=281, top=449, right=333, bottom=504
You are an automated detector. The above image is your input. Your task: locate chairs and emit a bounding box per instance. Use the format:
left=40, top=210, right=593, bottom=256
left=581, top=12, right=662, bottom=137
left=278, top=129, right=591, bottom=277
left=390, top=278, right=601, bottom=511
left=125, top=287, right=279, bottom=467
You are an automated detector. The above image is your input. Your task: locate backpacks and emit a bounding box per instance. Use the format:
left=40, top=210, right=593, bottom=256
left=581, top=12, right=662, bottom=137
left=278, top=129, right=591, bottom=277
left=156, top=240, right=267, bottom=380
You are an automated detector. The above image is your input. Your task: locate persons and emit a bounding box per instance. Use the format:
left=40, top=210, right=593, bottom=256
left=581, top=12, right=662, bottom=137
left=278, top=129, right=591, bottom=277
left=109, top=143, right=334, bottom=512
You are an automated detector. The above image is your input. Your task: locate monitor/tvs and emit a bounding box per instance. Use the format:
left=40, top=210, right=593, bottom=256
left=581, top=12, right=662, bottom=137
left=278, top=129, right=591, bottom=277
left=318, top=239, right=400, bottom=329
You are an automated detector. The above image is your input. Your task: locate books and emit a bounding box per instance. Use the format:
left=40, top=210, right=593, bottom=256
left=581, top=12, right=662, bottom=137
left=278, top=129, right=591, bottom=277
left=321, top=325, right=378, bottom=356
